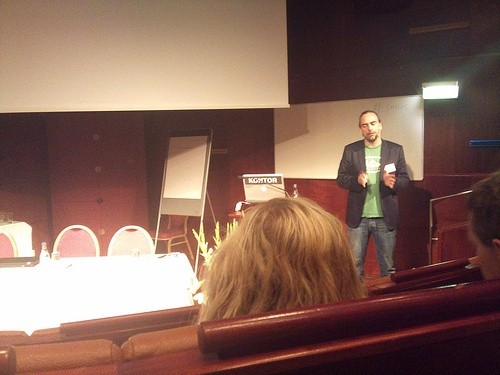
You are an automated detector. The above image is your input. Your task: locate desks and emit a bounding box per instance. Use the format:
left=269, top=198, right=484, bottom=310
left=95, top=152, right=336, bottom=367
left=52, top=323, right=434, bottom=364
left=1, top=252, right=200, bottom=336
left=0, top=220, right=32, bottom=257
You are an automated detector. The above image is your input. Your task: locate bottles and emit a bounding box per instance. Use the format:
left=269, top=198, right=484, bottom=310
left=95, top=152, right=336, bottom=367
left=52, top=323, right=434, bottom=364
left=40, top=242, right=50, bottom=270
left=292, top=184, right=299, bottom=198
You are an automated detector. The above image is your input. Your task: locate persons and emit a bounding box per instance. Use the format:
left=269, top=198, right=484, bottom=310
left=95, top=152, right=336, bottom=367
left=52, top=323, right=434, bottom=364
left=194, top=197, right=366, bottom=327
left=466, top=173, right=500, bottom=278
left=336, top=111, right=409, bottom=281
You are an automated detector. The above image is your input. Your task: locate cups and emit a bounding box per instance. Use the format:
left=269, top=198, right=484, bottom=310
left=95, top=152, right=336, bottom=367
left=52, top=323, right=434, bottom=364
left=51, top=252, right=59, bottom=265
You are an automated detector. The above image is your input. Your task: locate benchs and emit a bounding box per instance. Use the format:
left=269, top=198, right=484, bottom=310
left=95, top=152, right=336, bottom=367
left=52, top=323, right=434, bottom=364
left=0, top=258, right=500, bottom=375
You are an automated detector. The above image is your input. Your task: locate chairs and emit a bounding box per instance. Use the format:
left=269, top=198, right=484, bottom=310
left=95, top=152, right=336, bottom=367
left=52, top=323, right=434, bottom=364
left=149, top=215, right=195, bottom=259
left=107, top=225, right=155, bottom=256
left=0, top=233, right=18, bottom=258
left=53, top=224, right=100, bottom=257
